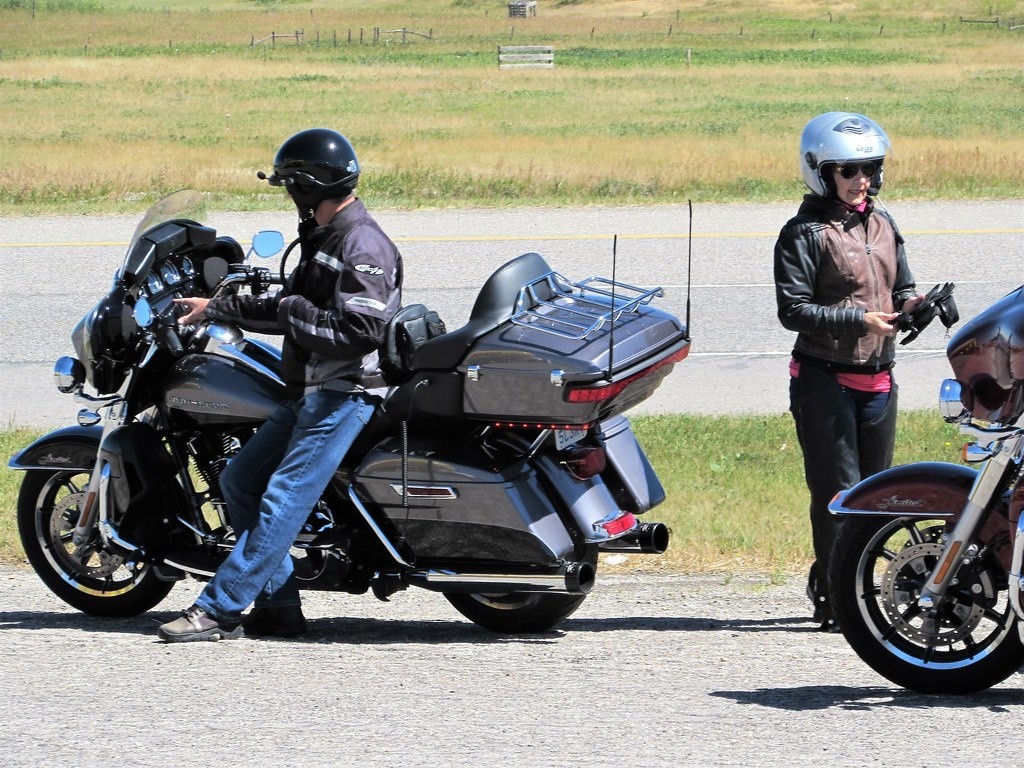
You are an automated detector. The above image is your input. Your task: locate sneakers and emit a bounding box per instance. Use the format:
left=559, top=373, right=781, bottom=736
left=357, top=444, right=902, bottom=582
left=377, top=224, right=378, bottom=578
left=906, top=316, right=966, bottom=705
left=238, top=608, right=307, bottom=638
left=158, top=605, right=244, bottom=641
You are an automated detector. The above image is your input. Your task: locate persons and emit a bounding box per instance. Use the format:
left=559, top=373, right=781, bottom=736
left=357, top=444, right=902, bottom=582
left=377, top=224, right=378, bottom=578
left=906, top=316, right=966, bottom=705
left=774, top=112, right=936, bottom=632
left=157, top=129, right=405, bottom=642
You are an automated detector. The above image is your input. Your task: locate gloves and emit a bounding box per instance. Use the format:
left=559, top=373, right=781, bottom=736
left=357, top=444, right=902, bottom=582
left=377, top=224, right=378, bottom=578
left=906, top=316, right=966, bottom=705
left=900, top=296, right=960, bottom=346
left=894, top=280, right=957, bottom=324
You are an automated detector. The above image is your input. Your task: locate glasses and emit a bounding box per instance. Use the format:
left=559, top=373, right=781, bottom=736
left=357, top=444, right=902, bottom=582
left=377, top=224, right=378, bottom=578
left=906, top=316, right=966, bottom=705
left=830, top=162, right=880, bottom=179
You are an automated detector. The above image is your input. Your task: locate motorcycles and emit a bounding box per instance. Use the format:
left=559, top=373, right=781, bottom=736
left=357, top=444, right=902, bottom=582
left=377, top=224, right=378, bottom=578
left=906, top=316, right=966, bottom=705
left=817, top=251, right=1023, bottom=696
left=9, top=182, right=693, bottom=629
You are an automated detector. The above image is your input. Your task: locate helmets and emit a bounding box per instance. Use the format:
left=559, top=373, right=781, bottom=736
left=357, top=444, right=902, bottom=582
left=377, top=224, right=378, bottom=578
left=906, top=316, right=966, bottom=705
left=268, top=129, right=359, bottom=212
left=800, top=113, right=892, bottom=198
